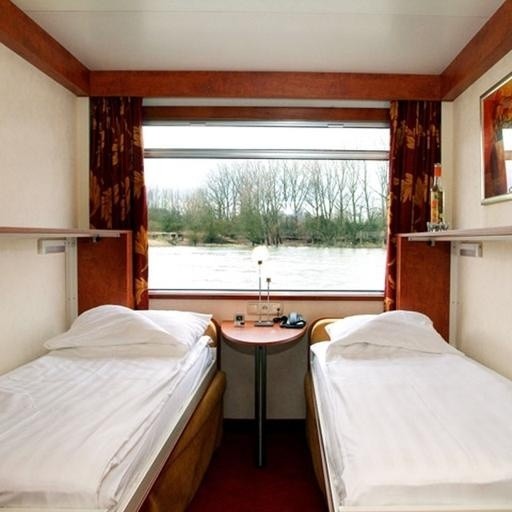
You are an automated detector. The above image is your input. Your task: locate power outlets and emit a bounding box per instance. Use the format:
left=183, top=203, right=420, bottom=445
left=247, top=302, right=283, bottom=314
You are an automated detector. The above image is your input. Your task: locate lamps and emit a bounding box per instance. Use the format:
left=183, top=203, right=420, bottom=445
left=252, top=246, right=276, bottom=326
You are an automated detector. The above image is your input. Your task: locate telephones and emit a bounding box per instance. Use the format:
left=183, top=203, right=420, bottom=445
left=279, top=312, right=304, bottom=328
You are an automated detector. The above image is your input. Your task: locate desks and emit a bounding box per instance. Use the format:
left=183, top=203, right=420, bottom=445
left=221, top=322, right=306, bottom=469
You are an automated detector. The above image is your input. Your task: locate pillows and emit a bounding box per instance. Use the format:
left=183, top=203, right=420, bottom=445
left=326, top=311, right=457, bottom=354
left=43, top=304, right=210, bottom=354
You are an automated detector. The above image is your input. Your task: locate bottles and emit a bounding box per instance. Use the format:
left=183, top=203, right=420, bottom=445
left=428, top=162, right=444, bottom=231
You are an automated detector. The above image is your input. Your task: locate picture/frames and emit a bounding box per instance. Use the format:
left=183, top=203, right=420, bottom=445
left=480, top=71, right=512, bottom=206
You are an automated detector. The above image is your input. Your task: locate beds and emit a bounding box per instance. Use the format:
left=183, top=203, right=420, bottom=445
left=304, top=317, right=511, bottom=512
left=0, top=318, right=225, bottom=512
